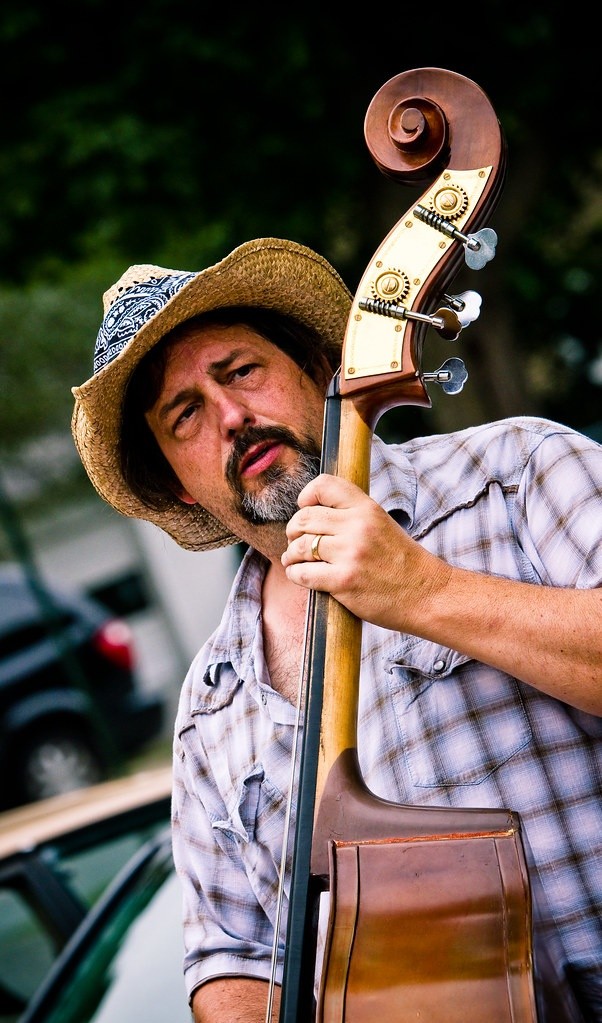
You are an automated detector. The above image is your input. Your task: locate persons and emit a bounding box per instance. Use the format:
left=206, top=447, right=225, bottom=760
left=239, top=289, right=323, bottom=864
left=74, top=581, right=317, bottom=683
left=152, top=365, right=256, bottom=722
left=70, top=236, right=602, bottom=1023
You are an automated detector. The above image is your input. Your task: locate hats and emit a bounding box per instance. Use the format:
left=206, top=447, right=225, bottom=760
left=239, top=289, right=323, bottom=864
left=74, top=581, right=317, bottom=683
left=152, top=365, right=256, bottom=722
left=69, top=236, right=355, bottom=553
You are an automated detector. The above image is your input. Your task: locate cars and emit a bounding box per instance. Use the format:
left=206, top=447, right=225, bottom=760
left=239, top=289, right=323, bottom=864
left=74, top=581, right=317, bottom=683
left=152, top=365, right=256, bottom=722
left=0, top=767, right=194, bottom=1023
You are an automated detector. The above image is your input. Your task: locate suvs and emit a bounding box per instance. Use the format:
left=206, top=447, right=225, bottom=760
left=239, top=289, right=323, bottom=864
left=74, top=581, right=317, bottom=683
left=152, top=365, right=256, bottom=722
left=0, top=565, right=173, bottom=816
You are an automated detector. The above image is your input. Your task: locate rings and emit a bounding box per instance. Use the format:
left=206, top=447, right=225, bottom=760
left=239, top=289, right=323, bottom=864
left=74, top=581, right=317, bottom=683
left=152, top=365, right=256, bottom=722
left=311, top=534, right=324, bottom=561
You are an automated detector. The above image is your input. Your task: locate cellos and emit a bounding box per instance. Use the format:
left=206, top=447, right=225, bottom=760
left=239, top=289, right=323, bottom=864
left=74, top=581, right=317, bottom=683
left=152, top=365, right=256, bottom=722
left=258, top=62, right=588, bottom=1023
